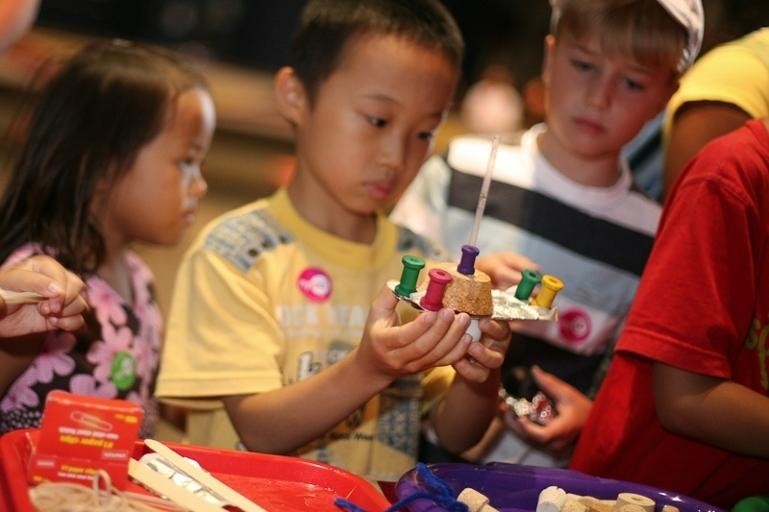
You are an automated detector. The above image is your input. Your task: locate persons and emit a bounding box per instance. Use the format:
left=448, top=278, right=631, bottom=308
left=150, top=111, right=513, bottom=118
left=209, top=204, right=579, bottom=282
left=392, top=3, right=765, bottom=509
left=153, top=0, right=512, bottom=477
left=1, top=38, right=217, bottom=440
left=1, top=254, right=89, bottom=401
